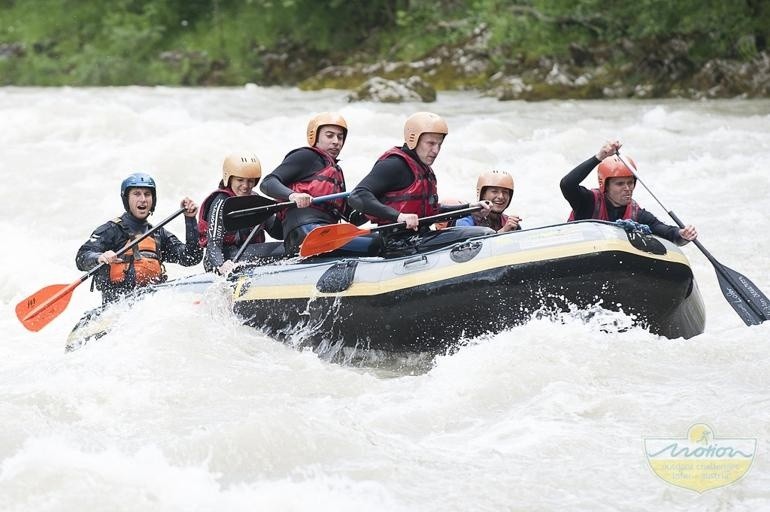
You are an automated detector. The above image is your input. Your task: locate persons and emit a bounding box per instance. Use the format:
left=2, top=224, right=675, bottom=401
left=259, top=112, right=379, bottom=256
left=559, top=138, right=699, bottom=248
left=348, top=111, right=496, bottom=260
left=199, top=149, right=282, bottom=277
left=455, top=169, right=522, bottom=239
left=75, top=172, right=204, bottom=308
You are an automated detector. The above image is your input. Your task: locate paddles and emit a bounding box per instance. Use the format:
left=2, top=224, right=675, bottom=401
left=301, top=203, right=487, bottom=258
left=221, top=191, right=353, bottom=233
left=14, top=207, right=188, bottom=332
left=614, top=150, right=769, bottom=328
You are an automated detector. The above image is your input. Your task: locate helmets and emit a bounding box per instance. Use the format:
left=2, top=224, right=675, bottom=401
left=598, top=155, right=637, bottom=192
left=121, top=173, right=155, bottom=199
left=306, top=112, right=348, bottom=147
left=403, top=110, right=448, bottom=150
left=477, top=169, right=514, bottom=203
left=223, top=148, right=261, bottom=187
